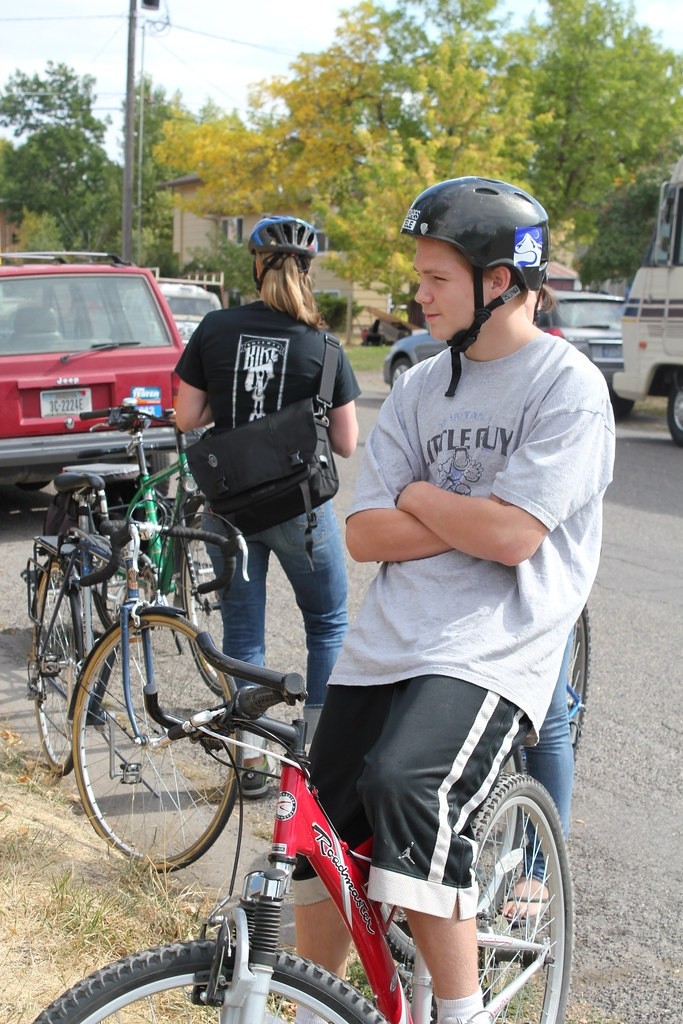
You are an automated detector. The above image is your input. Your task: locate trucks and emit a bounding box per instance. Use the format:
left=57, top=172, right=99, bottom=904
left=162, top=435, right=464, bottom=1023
left=613, top=149, right=682, bottom=443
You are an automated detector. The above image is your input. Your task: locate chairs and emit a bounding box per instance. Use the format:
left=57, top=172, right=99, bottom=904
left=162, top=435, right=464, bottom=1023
left=5, top=305, right=63, bottom=351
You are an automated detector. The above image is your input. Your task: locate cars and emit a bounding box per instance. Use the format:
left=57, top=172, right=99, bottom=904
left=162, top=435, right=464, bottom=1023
left=123, top=281, right=222, bottom=353
left=0, top=248, right=185, bottom=505
left=381, top=290, right=634, bottom=423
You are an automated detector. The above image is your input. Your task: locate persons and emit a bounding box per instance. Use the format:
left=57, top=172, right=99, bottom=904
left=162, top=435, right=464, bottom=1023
left=166, top=214, right=361, bottom=802
left=288, top=175, right=614, bottom=1024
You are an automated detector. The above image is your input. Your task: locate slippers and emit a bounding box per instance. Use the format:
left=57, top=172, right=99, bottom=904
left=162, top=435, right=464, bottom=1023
left=497, top=897, right=549, bottom=926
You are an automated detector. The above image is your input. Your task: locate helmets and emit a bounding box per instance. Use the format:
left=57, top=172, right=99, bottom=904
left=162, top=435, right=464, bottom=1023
left=400, top=176, right=550, bottom=291
left=248, top=216, right=318, bottom=258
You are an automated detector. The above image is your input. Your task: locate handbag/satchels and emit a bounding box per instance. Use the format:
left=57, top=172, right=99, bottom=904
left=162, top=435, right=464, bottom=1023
left=185, top=396, right=340, bottom=537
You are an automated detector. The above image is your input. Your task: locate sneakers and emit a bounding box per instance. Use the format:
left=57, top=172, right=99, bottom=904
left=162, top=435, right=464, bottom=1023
left=241, top=754, right=277, bottom=799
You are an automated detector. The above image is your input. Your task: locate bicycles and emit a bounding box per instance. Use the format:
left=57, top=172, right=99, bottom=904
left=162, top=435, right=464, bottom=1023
left=364, top=601, right=597, bottom=966
left=19, top=472, right=246, bottom=874
left=41, top=407, right=228, bottom=699
left=24, top=633, right=578, bottom=1024
